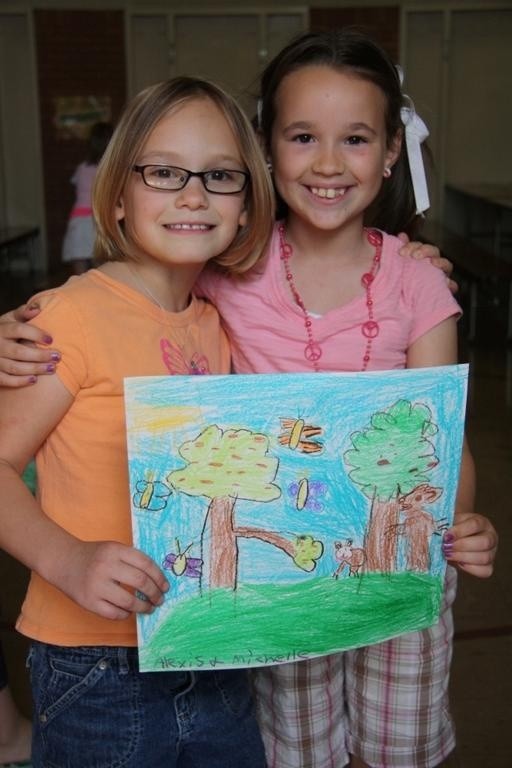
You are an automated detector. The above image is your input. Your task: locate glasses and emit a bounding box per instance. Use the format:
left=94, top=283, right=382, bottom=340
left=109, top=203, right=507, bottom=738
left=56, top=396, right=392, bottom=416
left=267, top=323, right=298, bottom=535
left=131, top=162, right=251, bottom=195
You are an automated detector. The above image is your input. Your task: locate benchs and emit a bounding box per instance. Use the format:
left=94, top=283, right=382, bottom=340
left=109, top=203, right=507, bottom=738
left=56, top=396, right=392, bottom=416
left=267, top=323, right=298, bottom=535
left=417, top=217, right=512, bottom=343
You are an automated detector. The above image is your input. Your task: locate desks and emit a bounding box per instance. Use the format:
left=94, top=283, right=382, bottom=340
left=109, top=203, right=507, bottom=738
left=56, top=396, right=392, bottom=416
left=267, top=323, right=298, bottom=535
left=426, top=175, right=512, bottom=280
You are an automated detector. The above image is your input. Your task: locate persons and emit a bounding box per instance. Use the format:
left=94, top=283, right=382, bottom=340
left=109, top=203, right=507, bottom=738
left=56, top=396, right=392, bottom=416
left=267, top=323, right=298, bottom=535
left=1, top=32, right=500, bottom=767
